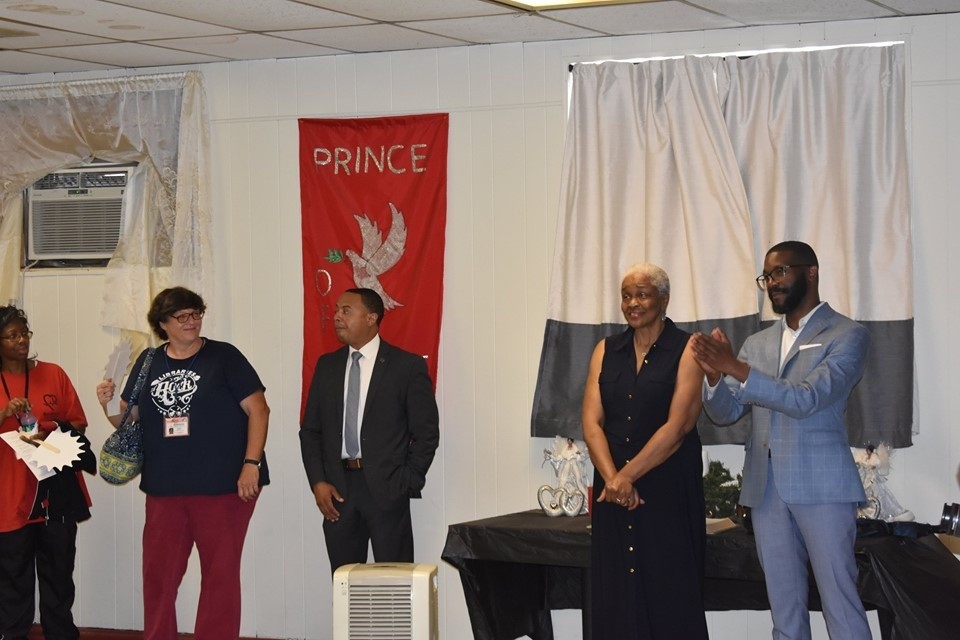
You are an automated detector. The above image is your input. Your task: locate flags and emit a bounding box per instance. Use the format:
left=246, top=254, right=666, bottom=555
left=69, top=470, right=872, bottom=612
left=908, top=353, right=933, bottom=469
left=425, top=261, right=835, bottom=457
left=297, top=109, right=449, bottom=449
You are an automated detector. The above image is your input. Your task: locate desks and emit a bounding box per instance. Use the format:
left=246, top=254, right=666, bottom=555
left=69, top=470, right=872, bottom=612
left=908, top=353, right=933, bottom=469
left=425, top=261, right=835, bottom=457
left=440, top=507, right=960, bottom=640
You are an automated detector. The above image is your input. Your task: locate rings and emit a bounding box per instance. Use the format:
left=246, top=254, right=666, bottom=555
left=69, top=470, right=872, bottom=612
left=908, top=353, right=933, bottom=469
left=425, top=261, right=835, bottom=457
left=616, top=498, right=621, bottom=502
left=627, top=498, right=631, bottom=501
left=103, top=387, right=106, bottom=394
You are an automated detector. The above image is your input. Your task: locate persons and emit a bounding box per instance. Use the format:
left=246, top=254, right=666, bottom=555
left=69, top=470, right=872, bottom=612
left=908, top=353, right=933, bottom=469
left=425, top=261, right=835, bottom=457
left=0, top=304, right=92, bottom=640
left=689, top=240, right=872, bottom=640
left=856, top=445, right=881, bottom=498
left=301, top=287, right=440, bottom=576
left=98, top=286, right=270, bottom=640
left=557, top=437, right=581, bottom=491
left=579, top=264, right=710, bottom=640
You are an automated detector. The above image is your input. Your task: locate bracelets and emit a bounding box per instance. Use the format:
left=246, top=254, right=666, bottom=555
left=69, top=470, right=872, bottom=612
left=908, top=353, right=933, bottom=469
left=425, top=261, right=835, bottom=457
left=243, top=458, right=262, bottom=469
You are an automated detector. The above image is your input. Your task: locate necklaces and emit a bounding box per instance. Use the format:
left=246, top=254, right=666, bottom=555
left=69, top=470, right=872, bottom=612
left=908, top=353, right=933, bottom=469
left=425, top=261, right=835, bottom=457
left=641, top=352, right=647, bottom=356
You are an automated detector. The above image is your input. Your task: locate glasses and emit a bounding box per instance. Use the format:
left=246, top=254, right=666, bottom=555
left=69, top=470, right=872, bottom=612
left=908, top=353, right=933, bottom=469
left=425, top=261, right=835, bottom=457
left=1, top=330, right=33, bottom=342
left=756, top=263, right=811, bottom=290
left=171, top=308, right=205, bottom=323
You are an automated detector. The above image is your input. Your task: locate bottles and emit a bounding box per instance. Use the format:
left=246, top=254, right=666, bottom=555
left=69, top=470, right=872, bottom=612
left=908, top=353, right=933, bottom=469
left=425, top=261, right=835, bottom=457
left=20, top=405, right=37, bottom=432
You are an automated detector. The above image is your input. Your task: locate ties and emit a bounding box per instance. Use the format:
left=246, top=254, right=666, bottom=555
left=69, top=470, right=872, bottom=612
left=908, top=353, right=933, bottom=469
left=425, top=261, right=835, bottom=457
left=345, top=351, right=363, bottom=460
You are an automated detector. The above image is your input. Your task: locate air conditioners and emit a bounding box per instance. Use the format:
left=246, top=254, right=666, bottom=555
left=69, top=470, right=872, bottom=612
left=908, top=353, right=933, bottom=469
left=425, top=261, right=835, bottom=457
left=26, top=165, right=141, bottom=262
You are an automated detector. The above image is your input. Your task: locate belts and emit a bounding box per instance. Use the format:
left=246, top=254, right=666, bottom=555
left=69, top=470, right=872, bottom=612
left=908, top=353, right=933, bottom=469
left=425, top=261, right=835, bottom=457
left=346, top=460, right=364, bottom=468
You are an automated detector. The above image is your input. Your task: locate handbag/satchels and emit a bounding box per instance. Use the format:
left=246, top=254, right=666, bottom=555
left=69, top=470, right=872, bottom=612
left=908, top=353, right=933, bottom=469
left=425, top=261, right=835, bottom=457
left=99, top=424, right=142, bottom=485
